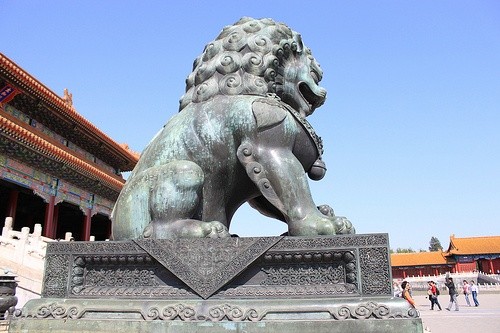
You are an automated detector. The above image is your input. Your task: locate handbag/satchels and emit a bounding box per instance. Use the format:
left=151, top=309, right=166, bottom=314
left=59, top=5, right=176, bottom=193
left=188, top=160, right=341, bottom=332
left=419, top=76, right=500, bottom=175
left=431, top=294, right=437, bottom=299
left=453, top=288, right=459, bottom=297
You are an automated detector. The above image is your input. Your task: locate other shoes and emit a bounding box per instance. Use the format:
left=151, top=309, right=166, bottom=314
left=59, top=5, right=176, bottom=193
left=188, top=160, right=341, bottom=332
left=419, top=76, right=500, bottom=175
left=445, top=307, right=459, bottom=311
left=425, top=326, right=432, bottom=333
left=430, top=308, right=443, bottom=311
left=465, top=304, right=471, bottom=306
left=475, top=304, right=478, bottom=306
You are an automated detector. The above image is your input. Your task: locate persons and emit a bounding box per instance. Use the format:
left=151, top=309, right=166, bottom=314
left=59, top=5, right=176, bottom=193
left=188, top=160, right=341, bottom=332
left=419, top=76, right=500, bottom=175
left=445, top=277, right=459, bottom=311
left=427, top=281, right=442, bottom=311
left=463, top=280, right=471, bottom=307
left=471, top=280, right=480, bottom=307
left=401, top=281, right=415, bottom=308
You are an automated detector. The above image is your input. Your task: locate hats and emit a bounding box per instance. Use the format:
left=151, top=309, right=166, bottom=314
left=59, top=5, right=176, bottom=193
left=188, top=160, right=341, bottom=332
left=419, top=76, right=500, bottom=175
left=402, top=281, right=409, bottom=289
left=448, top=278, right=452, bottom=281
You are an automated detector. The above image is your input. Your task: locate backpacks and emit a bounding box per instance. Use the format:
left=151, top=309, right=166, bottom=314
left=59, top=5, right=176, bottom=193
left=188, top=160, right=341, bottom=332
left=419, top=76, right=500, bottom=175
left=395, top=290, right=409, bottom=299
left=432, top=286, right=440, bottom=295
